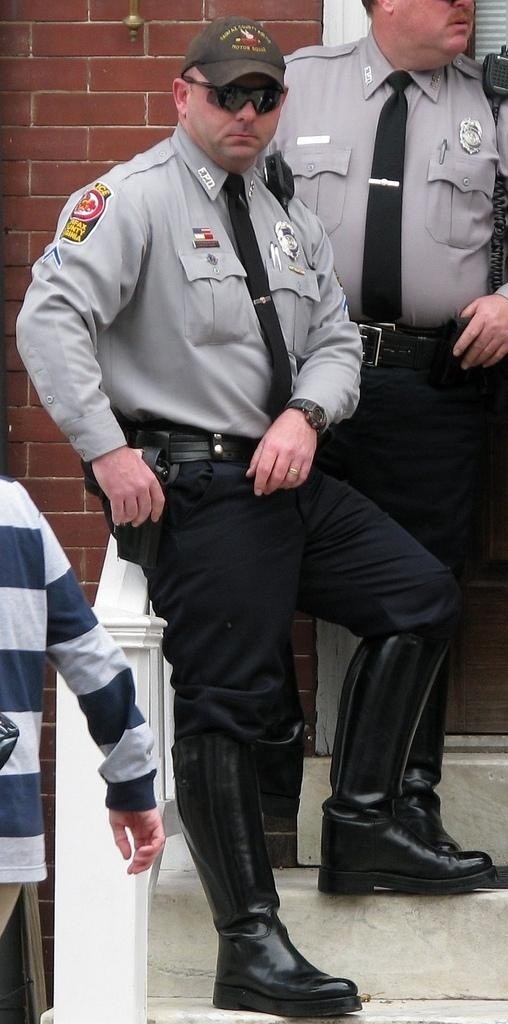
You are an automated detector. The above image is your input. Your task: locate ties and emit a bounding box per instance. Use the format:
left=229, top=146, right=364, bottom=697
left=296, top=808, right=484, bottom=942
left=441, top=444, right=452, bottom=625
left=360, top=71, right=415, bottom=324
left=222, top=172, right=293, bottom=423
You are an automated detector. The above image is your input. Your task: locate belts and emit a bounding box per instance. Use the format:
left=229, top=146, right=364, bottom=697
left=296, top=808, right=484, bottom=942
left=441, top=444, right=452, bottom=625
left=354, top=327, right=458, bottom=372
left=128, top=426, right=263, bottom=473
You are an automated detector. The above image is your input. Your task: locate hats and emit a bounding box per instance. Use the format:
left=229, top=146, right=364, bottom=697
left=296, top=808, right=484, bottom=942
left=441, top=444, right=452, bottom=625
left=184, top=14, right=288, bottom=90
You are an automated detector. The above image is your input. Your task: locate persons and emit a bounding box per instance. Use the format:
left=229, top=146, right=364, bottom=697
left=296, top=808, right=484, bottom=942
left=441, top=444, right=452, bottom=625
left=0, top=477, right=166, bottom=937
left=16, top=16, right=497, bottom=1018
left=256, top=0, right=508, bottom=857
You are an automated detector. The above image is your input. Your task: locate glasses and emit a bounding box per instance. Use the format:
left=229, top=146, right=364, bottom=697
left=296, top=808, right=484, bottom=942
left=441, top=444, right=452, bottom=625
left=185, top=75, right=283, bottom=114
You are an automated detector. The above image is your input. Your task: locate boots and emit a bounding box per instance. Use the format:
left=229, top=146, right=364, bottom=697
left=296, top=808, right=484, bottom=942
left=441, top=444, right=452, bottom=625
left=167, top=730, right=363, bottom=1020
left=395, top=649, right=462, bottom=856
left=319, top=633, right=495, bottom=895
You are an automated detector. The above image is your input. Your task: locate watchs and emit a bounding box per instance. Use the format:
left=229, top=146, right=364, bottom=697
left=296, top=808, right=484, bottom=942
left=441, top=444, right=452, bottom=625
left=286, top=400, right=328, bottom=435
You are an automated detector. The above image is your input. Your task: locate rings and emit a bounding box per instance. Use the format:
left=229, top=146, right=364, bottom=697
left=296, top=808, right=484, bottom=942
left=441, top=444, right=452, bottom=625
left=289, top=468, right=299, bottom=475
